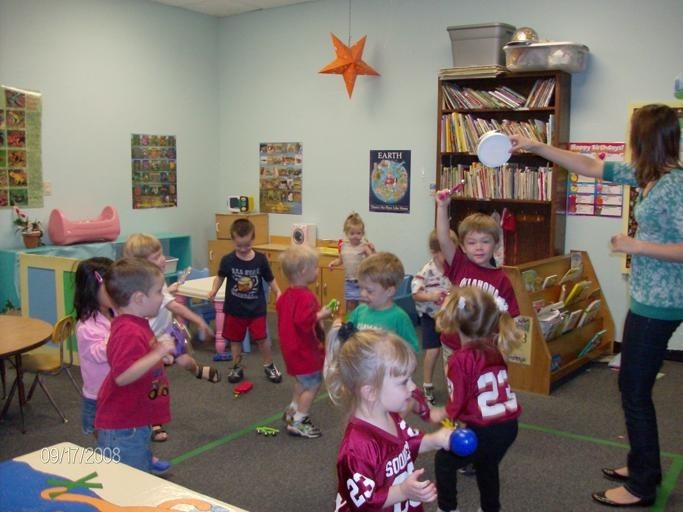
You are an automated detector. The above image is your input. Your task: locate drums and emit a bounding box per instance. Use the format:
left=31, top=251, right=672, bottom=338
left=476, top=131, right=512, bottom=170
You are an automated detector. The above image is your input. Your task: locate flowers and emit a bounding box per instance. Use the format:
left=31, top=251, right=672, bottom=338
left=12, top=206, right=44, bottom=234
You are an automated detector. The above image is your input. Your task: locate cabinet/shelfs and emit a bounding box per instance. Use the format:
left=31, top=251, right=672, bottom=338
left=0, top=232, right=193, bottom=315
left=430, top=69, right=571, bottom=264
left=254, top=236, right=346, bottom=322
left=500, top=250, right=616, bottom=396
left=207, top=212, right=270, bottom=275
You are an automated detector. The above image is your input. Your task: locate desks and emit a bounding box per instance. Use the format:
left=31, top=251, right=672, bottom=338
left=0, top=314, right=54, bottom=433
left=0, top=442, right=248, bottom=512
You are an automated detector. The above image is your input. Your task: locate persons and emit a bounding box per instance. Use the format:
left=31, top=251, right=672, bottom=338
left=411, top=228, right=458, bottom=401
left=208, top=218, right=282, bottom=383
left=506, top=102, right=683, bottom=507
left=431, top=285, right=522, bottom=511
left=274, top=246, right=340, bottom=438
left=324, top=318, right=454, bottom=511
left=72, top=234, right=220, bottom=474
left=435, top=189, right=523, bottom=475
left=334, top=251, right=420, bottom=352
left=329, top=211, right=376, bottom=315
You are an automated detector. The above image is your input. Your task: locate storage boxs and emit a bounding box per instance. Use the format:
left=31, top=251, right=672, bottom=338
left=447, top=22, right=516, bottom=68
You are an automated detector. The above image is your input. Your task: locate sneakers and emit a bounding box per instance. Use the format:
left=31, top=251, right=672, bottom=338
left=285, top=408, right=321, bottom=439
left=265, top=363, right=282, bottom=384
left=424, top=386, right=435, bottom=404
left=152, top=460, right=171, bottom=471
left=228, top=367, right=243, bottom=383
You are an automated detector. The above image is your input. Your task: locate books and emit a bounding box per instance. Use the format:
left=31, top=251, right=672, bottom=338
left=518, top=246, right=611, bottom=374
left=439, top=79, right=556, bottom=200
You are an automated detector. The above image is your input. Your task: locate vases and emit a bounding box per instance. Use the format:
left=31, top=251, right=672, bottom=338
left=21, top=232, right=40, bottom=249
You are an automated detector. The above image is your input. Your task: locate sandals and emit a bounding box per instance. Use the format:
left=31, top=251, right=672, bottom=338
left=195, top=364, right=220, bottom=383
left=151, top=424, right=168, bottom=441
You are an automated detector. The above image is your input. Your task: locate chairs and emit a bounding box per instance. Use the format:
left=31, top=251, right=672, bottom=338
left=8, top=316, right=81, bottom=428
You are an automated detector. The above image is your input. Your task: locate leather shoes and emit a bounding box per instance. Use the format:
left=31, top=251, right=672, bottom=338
left=591, top=487, right=656, bottom=507
left=602, top=468, right=630, bottom=480
